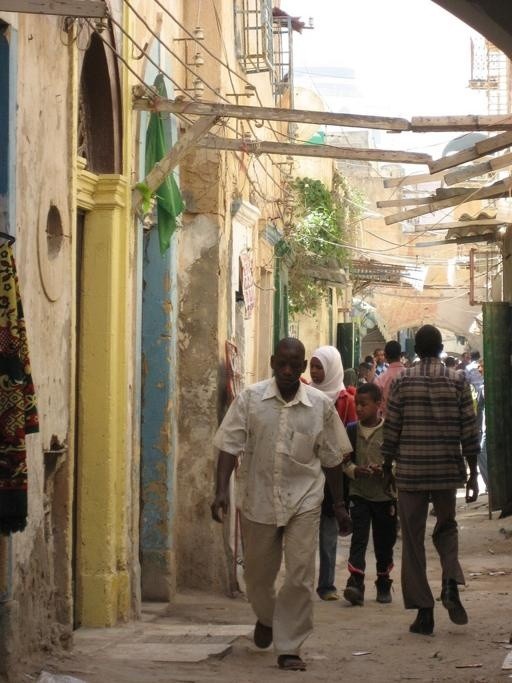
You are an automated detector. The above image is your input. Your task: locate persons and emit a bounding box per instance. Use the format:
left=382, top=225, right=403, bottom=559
left=210, top=338, right=356, bottom=673
left=339, top=341, right=488, bottom=392
left=375, top=322, right=479, bottom=633
left=337, top=378, right=399, bottom=605
left=473, top=385, right=489, bottom=492
left=303, top=342, right=360, bottom=602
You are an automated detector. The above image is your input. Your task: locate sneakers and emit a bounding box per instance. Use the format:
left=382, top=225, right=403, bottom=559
left=276, top=653, right=308, bottom=670
left=252, top=618, right=275, bottom=649
left=318, top=589, right=340, bottom=601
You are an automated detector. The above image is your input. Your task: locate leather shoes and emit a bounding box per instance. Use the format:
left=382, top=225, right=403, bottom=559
left=405, top=607, right=437, bottom=634
left=439, top=580, right=470, bottom=624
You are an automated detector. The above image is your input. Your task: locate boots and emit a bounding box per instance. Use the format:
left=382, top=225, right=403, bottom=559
left=341, top=570, right=366, bottom=606
left=373, top=572, right=396, bottom=603
